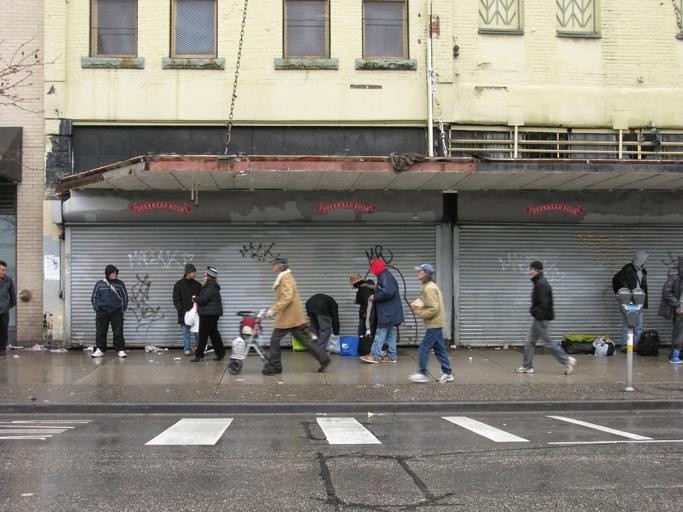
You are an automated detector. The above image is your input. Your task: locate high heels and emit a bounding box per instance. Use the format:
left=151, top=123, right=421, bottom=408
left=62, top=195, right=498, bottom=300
left=192, top=355, right=205, bottom=362
left=213, top=354, right=224, bottom=360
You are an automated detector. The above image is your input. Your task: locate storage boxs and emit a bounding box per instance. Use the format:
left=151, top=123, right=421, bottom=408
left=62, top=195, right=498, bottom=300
left=339, top=336, right=361, bottom=356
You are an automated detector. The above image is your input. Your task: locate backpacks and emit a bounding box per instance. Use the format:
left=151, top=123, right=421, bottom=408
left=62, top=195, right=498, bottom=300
left=637, top=330, right=661, bottom=356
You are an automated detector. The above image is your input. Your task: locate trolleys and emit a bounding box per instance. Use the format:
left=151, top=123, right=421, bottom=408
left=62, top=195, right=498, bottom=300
left=225, top=309, right=277, bottom=378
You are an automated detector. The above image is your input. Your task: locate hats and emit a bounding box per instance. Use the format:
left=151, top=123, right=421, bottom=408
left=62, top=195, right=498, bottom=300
left=352, top=274, right=363, bottom=285
left=185, top=264, right=196, bottom=273
left=416, top=263, right=434, bottom=274
left=271, top=257, right=287, bottom=263
left=207, top=268, right=217, bottom=278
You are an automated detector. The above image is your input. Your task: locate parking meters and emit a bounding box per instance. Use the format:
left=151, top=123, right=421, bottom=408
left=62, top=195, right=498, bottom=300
left=616, top=287, right=647, bottom=395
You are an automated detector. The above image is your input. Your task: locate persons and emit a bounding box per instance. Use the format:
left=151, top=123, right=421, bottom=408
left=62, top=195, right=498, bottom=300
left=89, top=264, right=129, bottom=357
left=610, top=250, right=646, bottom=353
left=350, top=272, right=378, bottom=340
left=261, top=257, right=330, bottom=374
left=408, top=263, right=455, bottom=383
left=171, top=264, right=206, bottom=355
left=657, top=257, right=683, bottom=364
left=304, top=294, right=339, bottom=354
left=514, top=261, right=578, bottom=375
left=188, top=267, right=223, bottom=361
left=360, top=259, right=404, bottom=364
left=0, top=259, right=15, bottom=354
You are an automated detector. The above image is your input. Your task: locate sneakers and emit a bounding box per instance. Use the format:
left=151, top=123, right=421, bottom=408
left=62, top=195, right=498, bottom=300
left=91, top=348, right=104, bottom=357
left=518, top=367, right=535, bottom=373
left=410, top=372, right=455, bottom=383
left=118, top=351, right=127, bottom=357
left=360, top=355, right=393, bottom=364
left=318, top=359, right=329, bottom=372
left=563, top=358, right=577, bottom=374
left=262, top=366, right=282, bottom=374
